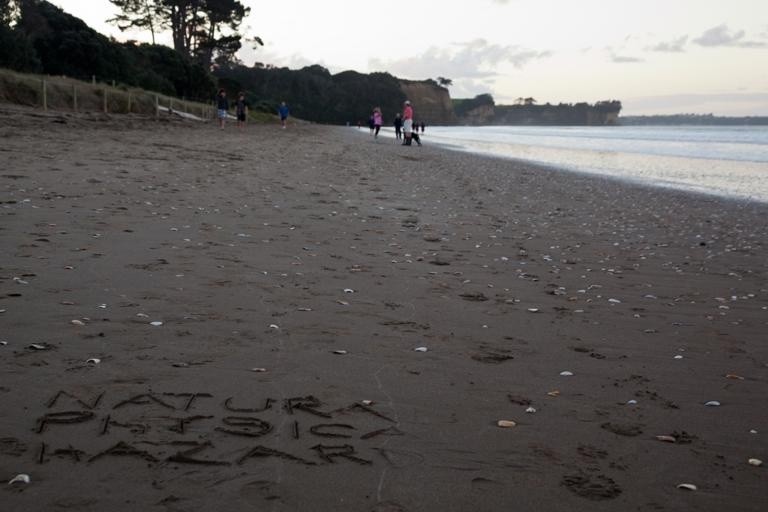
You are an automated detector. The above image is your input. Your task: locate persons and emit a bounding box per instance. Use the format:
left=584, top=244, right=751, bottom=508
left=217, top=88, right=229, bottom=130
left=278, top=101, right=290, bottom=130
left=368, top=100, right=425, bottom=145
left=235, top=91, right=246, bottom=128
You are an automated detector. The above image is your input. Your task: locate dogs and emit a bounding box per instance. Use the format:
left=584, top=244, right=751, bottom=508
left=402, top=128, right=421, bottom=146
